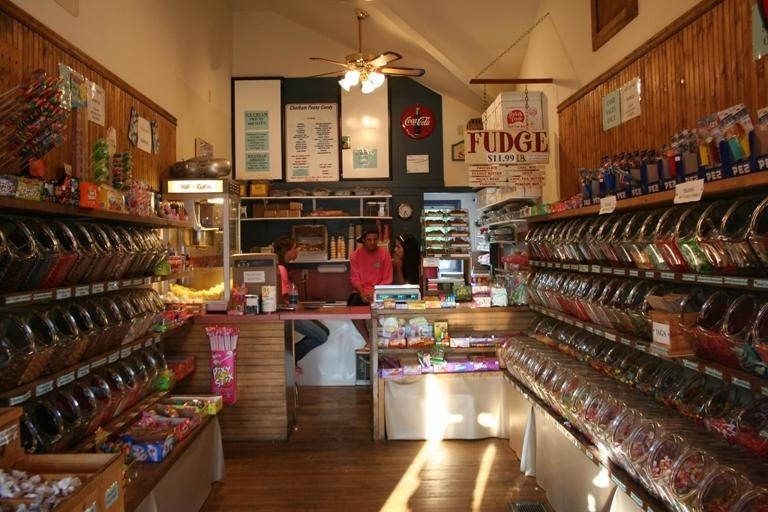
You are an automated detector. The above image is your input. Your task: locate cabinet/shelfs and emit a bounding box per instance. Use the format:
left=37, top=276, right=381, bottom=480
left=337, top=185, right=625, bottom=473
left=0, top=192, right=229, bottom=510
left=493, top=167, right=768, bottom=512
left=237, top=196, right=391, bottom=268
left=365, top=299, right=531, bottom=444
left=474, top=195, right=531, bottom=275
left=420, top=208, right=471, bottom=262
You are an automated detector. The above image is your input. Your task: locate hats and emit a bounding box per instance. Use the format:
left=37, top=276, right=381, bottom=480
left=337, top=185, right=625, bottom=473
left=356, top=222, right=379, bottom=244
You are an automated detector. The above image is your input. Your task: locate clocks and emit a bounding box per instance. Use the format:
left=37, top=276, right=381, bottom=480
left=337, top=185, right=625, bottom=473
left=396, top=201, right=415, bottom=219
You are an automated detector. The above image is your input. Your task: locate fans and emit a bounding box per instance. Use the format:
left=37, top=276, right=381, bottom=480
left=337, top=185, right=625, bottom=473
left=301, top=5, right=428, bottom=83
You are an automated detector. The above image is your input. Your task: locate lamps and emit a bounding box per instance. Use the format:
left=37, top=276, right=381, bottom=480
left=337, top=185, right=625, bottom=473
left=333, top=69, right=388, bottom=94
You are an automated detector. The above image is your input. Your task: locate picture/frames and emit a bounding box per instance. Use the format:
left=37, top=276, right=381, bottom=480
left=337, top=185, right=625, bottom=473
left=282, top=99, right=341, bottom=183
left=229, top=74, right=286, bottom=182
left=338, top=76, right=394, bottom=182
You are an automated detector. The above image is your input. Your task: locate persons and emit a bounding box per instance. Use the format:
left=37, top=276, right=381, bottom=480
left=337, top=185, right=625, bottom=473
left=274, top=238, right=329, bottom=373
left=393, top=233, right=420, bottom=285
left=345, top=223, right=394, bottom=353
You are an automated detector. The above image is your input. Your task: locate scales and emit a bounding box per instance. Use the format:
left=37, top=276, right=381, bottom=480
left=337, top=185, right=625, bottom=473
left=373, top=285, right=421, bottom=303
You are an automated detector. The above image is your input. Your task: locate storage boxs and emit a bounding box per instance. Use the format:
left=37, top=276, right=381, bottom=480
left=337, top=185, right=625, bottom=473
left=0, top=442, right=128, bottom=510
left=480, top=90, right=545, bottom=132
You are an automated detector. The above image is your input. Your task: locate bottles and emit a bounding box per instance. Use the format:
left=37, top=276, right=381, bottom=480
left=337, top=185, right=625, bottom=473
left=540, top=107, right=765, bottom=217
left=0, top=210, right=173, bottom=453
left=288, top=288, right=298, bottom=310
left=497, top=194, right=767, bottom=512
left=375, top=220, right=391, bottom=243
left=412, top=108, right=424, bottom=137
left=260, top=285, right=276, bottom=315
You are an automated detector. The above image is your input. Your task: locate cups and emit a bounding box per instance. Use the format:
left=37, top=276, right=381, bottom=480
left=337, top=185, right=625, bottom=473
left=331, top=236, right=346, bottom=260
